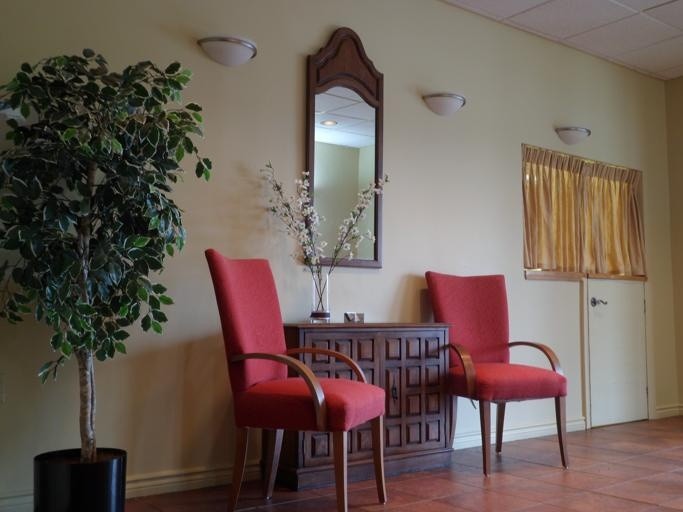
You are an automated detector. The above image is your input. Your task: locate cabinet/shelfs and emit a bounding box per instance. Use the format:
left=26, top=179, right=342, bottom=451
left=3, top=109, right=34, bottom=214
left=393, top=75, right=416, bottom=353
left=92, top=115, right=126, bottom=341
left=262, top=324, right=454, bottom=489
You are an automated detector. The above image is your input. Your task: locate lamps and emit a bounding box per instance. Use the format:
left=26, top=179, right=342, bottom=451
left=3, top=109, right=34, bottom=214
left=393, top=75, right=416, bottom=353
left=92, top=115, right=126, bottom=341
left=198, top=37, right=258, bottom=66
left=556, top=127, right=590, bottom=145
left=423, top=93, right=466, bottom=115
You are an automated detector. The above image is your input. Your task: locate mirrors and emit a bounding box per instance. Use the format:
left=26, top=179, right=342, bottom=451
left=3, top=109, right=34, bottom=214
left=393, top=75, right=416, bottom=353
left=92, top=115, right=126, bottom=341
left=306, top=27, right=384, bottom=269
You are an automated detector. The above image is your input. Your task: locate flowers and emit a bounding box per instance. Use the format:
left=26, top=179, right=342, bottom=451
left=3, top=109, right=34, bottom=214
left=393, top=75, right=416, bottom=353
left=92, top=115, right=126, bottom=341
left=262, top=162, right=389, bottom=311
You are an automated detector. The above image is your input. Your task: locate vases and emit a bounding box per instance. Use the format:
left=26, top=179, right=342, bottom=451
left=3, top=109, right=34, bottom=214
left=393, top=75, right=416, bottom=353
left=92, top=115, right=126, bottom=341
left=309, top=271, right=331, bottom=323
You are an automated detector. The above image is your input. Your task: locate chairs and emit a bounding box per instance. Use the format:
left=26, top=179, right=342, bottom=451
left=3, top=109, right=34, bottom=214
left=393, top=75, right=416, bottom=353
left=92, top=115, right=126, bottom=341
left=426, top=272, right=569, bottom=474
left=206, top=249, right=386, bottom=511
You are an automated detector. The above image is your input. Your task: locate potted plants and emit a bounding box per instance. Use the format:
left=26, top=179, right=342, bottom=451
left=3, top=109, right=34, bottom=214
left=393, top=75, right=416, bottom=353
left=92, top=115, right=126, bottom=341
left=0, top=48, right=212, bottom=512
